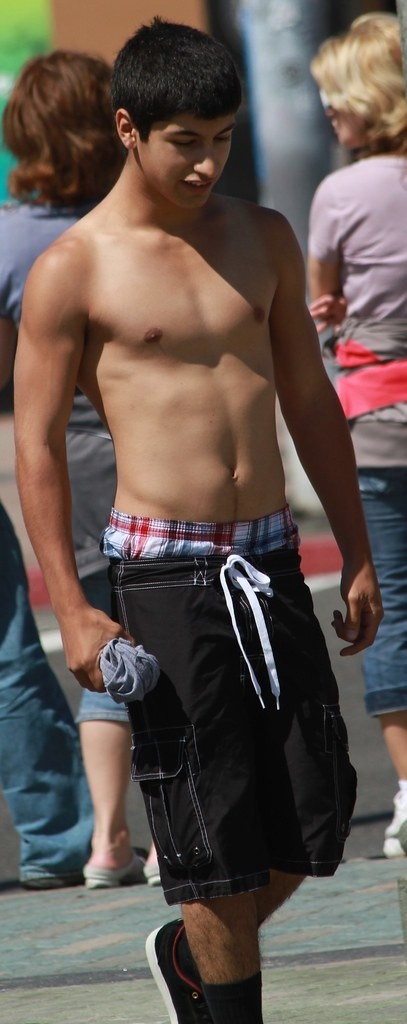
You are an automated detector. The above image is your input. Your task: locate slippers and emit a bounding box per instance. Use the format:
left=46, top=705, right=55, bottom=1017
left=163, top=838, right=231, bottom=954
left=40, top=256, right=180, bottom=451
left=143, top=862, right=160, bottom=886
left=82, top=849, right=147, bottom=888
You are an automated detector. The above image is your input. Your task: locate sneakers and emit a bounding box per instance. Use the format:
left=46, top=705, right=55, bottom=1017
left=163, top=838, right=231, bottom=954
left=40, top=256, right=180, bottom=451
left=145, top=918, right=213, bottom=1024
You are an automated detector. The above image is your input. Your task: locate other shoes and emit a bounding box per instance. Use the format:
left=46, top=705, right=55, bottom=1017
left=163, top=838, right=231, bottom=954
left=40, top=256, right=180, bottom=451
left=384, top=791, right=407, bottom=860
left=20, top=876, right=86, bottom=890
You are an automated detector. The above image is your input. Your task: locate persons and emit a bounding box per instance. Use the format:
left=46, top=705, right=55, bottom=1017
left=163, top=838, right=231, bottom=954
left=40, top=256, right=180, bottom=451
left=10, top=16, right=383, bottom=1024
left=304, top=13, right=406, bottom=864
left=0, top=47, right=170, bottom=893
